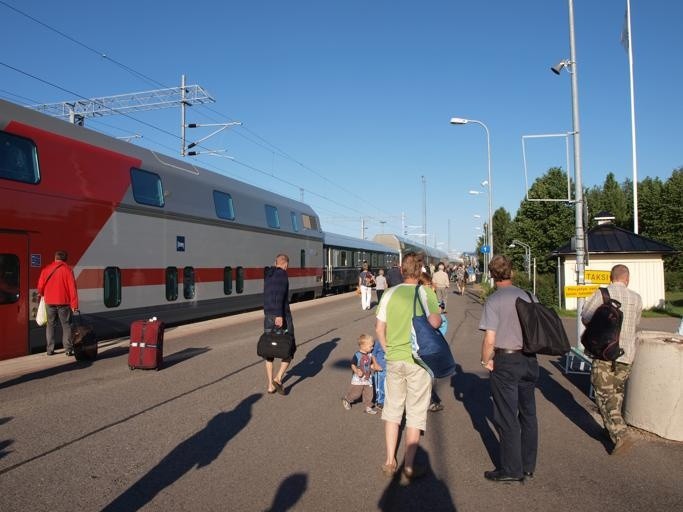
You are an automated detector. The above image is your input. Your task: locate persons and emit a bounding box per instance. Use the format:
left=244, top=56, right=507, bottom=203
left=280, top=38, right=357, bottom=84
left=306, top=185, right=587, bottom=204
left=38, top=251, right=78, bottom=355
left=375, top=252, right=442, bottom=478
left=342, top=262, right=479, bottom=415
left=264, top=255, right=296, bottom=395
left=580, top=265, right=643, bottom=454
left=479, top=255, right=540, bottom=486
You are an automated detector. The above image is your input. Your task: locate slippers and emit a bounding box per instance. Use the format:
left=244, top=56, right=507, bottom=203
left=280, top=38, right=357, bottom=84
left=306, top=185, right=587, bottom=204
left=268, top=388, right=276, bottom=393
left=272, top=380, right=285, bottom=395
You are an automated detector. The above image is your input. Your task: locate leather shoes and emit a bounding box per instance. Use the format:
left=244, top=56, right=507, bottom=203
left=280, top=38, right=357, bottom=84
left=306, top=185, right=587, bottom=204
left=611, top=433, right=642, bottom=455
left=527, top=472, right=533, bottom=477
left=483, top=471, right=525, bottom=486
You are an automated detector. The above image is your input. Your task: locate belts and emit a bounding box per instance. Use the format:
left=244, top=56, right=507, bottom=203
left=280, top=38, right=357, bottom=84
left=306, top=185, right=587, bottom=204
left=494, top=348, right=522, bottom=354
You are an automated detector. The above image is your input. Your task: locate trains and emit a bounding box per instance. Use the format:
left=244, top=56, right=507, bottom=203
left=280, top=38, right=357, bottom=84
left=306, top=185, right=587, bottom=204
left=0, top=94, right=447, bottom=366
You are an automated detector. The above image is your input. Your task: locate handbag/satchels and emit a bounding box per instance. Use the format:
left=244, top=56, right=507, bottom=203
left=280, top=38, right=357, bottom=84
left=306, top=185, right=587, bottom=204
left=411, top=315, right=457, bottom=378
left=516, top=296, right=570, bottom=355
left=257, top=323, right=296, bottom=358
left=365, top=271, right=375, bottom=287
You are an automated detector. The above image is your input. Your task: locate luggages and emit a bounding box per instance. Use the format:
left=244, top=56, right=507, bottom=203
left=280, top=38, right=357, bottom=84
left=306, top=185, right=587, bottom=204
left=71, top=308, right=98, bottom=360
left=128, top=316, right=165, bottom=372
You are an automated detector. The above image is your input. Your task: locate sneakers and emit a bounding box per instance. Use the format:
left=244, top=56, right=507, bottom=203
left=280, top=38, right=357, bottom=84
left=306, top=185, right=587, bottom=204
left=341, top=394, right=354, bottom=410
left=400, top=468, right=411, bottom=484
left=382, top=462, right=398, bottom=477
left=364, top=408, right=377, bottom=415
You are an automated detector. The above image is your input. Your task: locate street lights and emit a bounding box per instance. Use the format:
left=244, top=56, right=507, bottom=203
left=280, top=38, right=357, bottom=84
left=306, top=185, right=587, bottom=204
left=449, top=117, right=495, bottom=294
left=420, top=175, right=428, bottom=249
left=507, top=240, right=531, bottom=281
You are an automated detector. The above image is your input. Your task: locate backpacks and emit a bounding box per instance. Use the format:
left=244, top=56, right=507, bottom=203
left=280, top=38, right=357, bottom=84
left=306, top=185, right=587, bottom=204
left=580, top=286, right=624, bottom=369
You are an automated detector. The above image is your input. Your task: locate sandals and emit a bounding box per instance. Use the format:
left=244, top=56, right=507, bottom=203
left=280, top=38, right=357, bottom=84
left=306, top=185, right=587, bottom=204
left=430, top=404, right=443, bottom=412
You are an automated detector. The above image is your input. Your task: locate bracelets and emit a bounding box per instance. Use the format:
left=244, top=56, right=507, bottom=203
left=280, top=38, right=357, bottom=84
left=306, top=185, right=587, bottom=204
left=481, top=361, right=488, bottom=367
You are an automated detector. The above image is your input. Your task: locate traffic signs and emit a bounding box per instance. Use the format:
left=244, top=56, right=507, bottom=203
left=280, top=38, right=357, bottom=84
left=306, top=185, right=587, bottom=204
left=561, top=285, right=600, bottom=298
left=581, top=268, right=612, bottom=284
